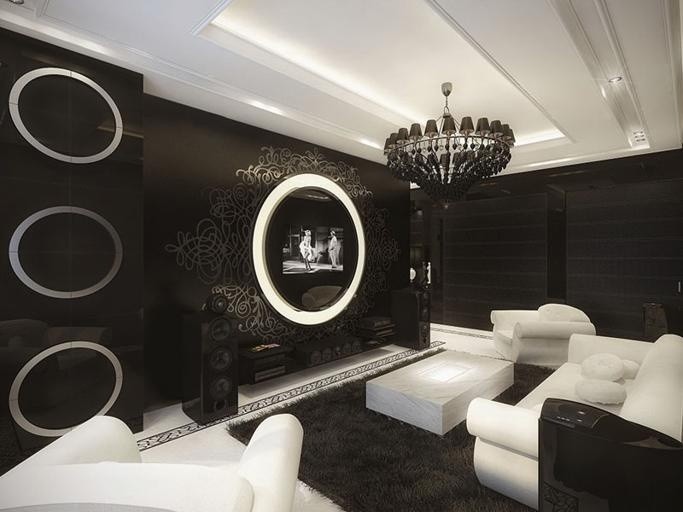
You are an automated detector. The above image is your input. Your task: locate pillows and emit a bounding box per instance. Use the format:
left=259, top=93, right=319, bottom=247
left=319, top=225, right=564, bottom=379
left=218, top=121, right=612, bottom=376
left=575, top=379, right=627, bottom=404
left=581, top=352, right=624, bottom=382
left=620, top=359, right=640, bottom=380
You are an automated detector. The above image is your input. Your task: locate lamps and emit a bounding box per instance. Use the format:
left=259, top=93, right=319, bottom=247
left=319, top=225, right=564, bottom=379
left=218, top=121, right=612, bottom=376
left=383, top=82, right=514, bottom=211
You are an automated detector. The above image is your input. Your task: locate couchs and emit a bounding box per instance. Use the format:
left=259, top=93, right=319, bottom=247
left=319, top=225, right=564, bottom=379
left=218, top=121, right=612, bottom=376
left=467, top=332, right=683, bottom=512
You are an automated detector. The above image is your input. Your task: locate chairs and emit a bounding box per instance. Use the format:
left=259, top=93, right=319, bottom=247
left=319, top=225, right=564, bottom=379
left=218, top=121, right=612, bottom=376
left=491, top=303, right=596, bottom=367
left=0, top=414, right=305, bottom=512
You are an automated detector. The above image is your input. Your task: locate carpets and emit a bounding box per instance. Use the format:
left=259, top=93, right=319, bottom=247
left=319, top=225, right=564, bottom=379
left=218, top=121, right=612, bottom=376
left=226, top=348, right=556, bottom=512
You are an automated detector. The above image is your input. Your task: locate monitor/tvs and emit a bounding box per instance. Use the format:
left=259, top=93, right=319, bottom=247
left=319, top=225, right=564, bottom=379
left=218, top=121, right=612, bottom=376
left=269, top=218, right=352, bottom=283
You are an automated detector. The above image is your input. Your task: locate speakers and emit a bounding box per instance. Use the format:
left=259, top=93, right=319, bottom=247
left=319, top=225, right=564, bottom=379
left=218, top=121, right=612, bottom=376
left=390, top=289, right=431, bottom=351
left=208, top=293, right=229, bottom=314
left=198, top=311, right=238, bottom=417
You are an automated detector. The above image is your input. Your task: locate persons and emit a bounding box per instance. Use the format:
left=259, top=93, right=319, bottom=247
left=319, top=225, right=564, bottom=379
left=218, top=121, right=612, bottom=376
left=326, top=230, right=339, bottom=268
left=299, top=229, right=318, bottom=271
